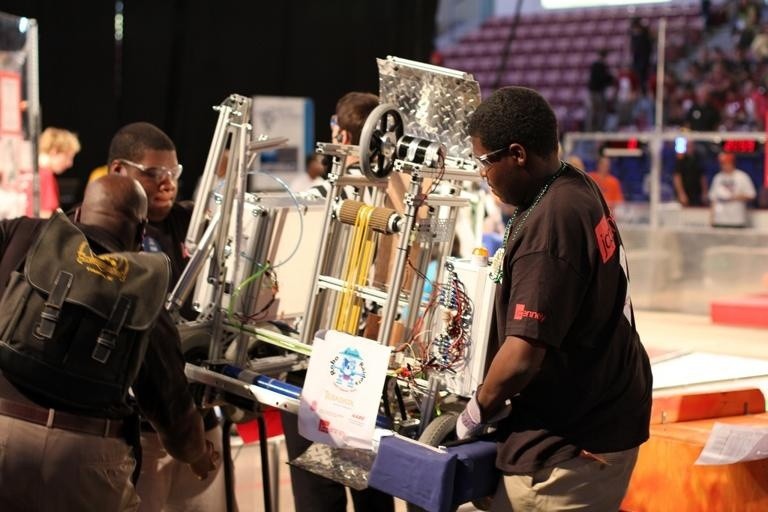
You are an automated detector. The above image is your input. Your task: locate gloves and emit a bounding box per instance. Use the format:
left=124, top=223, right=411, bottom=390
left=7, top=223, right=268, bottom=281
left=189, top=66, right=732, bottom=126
left=452, top=385, right=511, bottom=443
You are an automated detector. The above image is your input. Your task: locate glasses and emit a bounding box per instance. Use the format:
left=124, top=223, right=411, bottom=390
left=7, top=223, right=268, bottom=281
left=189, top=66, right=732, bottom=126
left=471, top=148, right=506, bottom=169
left=121, top=158, right=183, bottom=184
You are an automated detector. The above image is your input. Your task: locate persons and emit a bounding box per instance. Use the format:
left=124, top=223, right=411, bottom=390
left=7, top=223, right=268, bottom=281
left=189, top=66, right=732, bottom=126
left=0, top=174, right=221, bottom=511
left=106, top=119, right=223, bottom=512
left=454, top=86, right=652, bottom=510
left=25, top=126, right=82, bottom=223
left=306, top=91, right=380, bottom=178
left=587, top=0, right=767, bottom=229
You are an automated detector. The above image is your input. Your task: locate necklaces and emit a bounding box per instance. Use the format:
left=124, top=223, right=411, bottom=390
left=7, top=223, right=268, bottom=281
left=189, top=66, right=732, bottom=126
left=488, top=160, right=568, bottom=285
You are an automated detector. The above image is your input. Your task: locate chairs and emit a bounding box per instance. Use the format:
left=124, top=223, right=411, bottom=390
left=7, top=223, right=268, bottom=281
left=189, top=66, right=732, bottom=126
left=440, top=1, right=706, bottom=137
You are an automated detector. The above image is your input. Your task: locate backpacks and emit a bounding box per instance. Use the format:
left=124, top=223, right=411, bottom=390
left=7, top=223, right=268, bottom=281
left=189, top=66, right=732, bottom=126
left=1, top=206, right=176, bottom=421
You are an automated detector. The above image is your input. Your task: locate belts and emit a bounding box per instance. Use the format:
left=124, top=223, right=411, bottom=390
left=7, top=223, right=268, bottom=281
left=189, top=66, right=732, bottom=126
left=1, top=398, right=122, bottom=439
